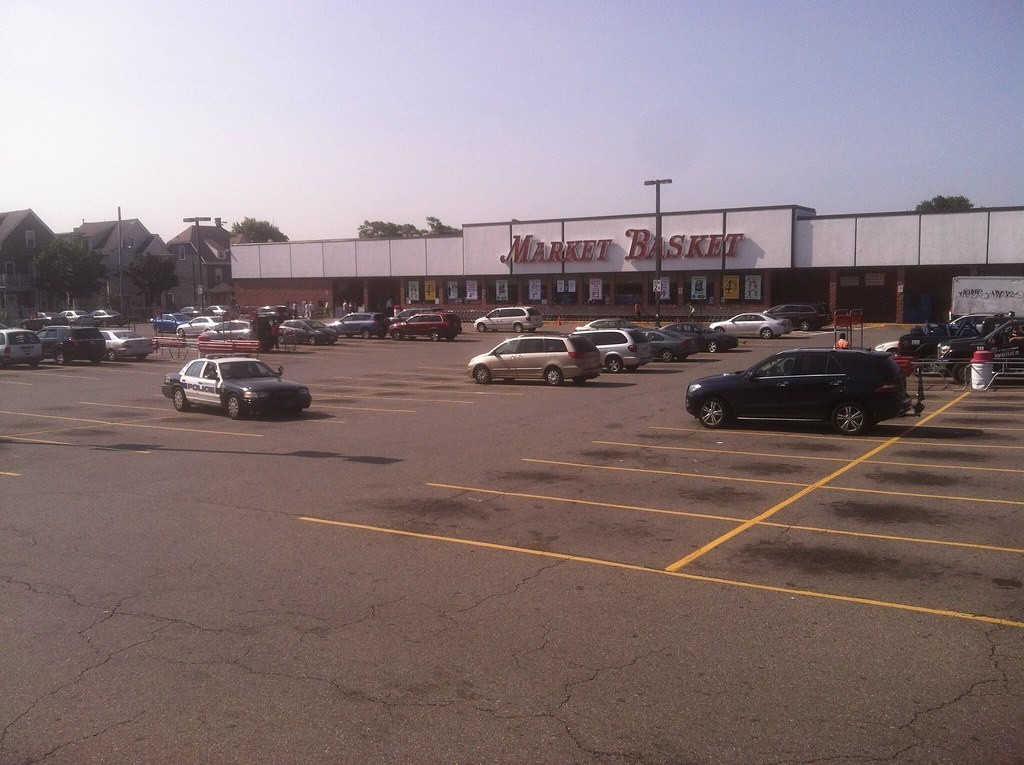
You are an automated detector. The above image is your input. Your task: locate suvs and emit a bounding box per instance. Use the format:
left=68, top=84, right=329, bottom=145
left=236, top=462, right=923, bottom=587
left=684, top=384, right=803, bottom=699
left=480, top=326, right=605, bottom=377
left=936, top=318, right=1024, bottom=385
left=764, top=302, right=832, bottom=331
left=388, top=306, right=444, bottom=327
left=198, top=320, right=257, bottom=341
left=36, top=323, right=108, bottom=367
left=685, top=347, right=912, bottom=438
left=174, top=316, right=224, bottom=338
left=467, top=333, right=603, bottom=386
left=0, top=327, right=45, bottom=370
left=250, top=306, right=292, bottom=325
left=568, top=328, right=652, bottom=372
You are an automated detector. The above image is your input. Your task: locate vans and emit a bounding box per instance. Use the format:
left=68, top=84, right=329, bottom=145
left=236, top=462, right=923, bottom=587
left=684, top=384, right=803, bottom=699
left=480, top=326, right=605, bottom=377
left=473, top=306, right=544, bottom=334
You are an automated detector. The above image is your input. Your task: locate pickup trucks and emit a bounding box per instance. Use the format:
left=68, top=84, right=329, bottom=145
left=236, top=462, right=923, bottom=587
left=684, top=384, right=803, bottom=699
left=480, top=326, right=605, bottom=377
left=899, top=316, right=1017, bottom=372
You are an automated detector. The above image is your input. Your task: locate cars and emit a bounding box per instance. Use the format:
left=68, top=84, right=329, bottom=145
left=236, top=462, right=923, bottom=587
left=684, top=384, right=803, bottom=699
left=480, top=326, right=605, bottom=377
left=944, top=313, right=1015, bottom=337
left=660, top=322, right=740, bottom=352
left=642, top=329, right=699, bottom=361
left=161, top=352, right=313, bottom=419
left=389, top=312, right=462, bottom=342
left=58, top=310, right=94, bottom=325
left=208, top=304, right=234, bottom=316
left=329, top=311, right=388, bottom=339
left=175, top=305, right=204, bottom=317
left=708, top=312, right=792, bottom=340
left=89, top=309, right=127, bottom=327
left=277, top=319, right=338, bottom=345
left=154, top=313, right=194, bottom=334
left=21, top=311, right=68, bottom=331
left=574, top=318, right=647, bottom=334
left=99, top=330, right=154, bottom=362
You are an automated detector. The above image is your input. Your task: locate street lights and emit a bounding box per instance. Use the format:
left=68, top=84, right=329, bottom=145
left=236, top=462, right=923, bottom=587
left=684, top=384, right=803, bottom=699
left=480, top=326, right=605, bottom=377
left=641, top=179, right=672, bottom=325
left=184, top=217, right=212, bottom=305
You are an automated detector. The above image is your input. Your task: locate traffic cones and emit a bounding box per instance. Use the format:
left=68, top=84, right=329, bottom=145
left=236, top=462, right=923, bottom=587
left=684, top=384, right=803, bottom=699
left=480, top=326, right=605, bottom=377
left=556, top=316, right=561, bottom=325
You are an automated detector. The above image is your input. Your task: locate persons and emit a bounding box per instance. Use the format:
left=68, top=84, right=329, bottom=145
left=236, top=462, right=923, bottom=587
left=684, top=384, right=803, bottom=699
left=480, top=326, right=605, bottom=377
left=270, top=322, right=280, bottom=352
left=285, top=295, right=356, bottom=318
left=780, top=359, right=794, bottom=376
left=386, top=298, right=392, bottom=313
left=687, top=302, right=694, bottom=322
left=834, top=333, right=849, bottom=349
left=635, top=302, right=642, bottom=320
left=1005, top=326, right=1023, bottom=343
